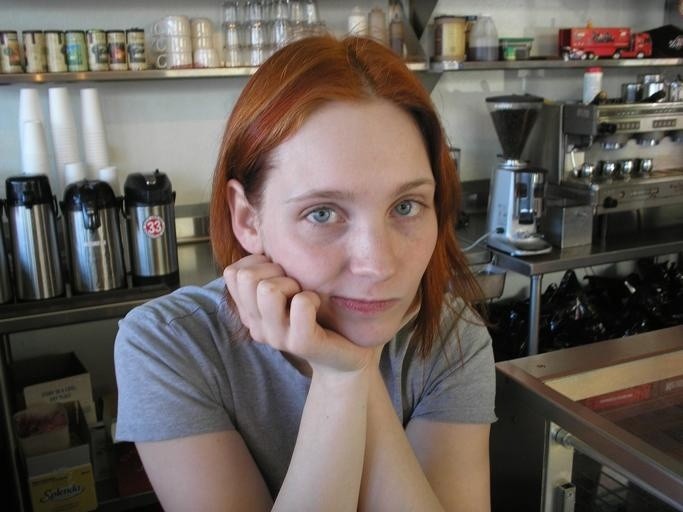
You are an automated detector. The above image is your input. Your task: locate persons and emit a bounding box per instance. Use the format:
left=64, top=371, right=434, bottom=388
left=112, top=35, right=496, bottom=512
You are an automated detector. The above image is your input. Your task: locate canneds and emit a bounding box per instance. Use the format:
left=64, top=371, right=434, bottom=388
left=23, top=31, right=47, bottom=73
left=126, top=29, right=147, bottom=70
left=0, top=30, right=24, bottom=73
left=107, top=30, right=129, bottom=71
left=65, top=30, right=89, bottom=72
left=85, top=29, right=110, bottom=71
left=44, top=30, right=68, bottom=72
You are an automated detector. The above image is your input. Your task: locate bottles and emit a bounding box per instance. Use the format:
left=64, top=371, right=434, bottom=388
left=583, top=67, right=603, bottom=107
left=1, top=176, right=180, bottom=301
left=621, top=73, right=683, bottom=103
left=347, top=3, right=406, bottom=61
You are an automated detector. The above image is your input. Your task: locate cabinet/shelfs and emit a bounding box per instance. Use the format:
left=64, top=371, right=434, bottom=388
left=4, top=51, right=680, bottom=512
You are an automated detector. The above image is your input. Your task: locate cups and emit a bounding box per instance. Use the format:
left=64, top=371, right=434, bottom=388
left=17, top=89, right=123, bottom=198
left=582, top=156, right=653, bottom=180
left=152, top=15, right=223, bottom=68
left=220, top=1, right=320, bottom=65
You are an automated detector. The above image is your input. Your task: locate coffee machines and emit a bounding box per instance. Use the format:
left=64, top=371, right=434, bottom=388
left=484, top=93, right=554, bottom=256
